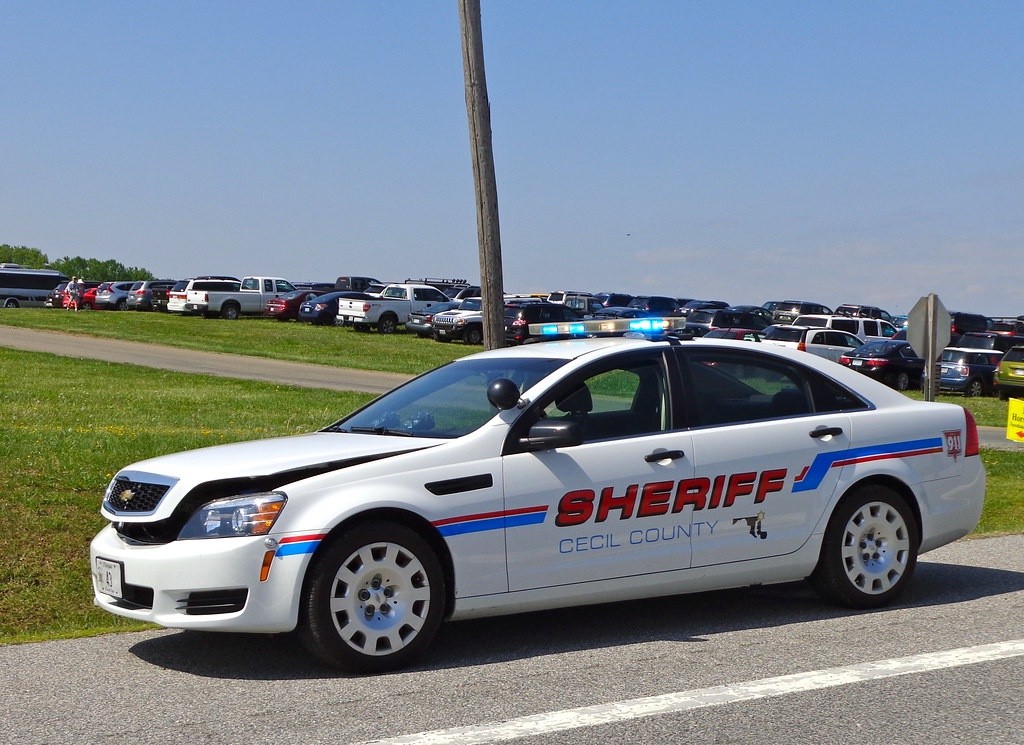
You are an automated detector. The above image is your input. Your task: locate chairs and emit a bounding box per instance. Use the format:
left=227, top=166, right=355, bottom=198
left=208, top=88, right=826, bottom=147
left=554, top=382, right=593, bottom=441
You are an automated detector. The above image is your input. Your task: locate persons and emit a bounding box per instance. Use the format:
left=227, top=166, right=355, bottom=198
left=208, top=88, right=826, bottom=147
left=67, top=276, right=80, bottom=312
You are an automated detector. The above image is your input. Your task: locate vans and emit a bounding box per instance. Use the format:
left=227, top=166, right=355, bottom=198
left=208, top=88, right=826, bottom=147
left=792, top=314, right=900, bottom=344
left=947, top=311, right=993, bottom=338
left=335, top=276, right=383, bottom=292
left=0, top=263, right=72, bottom=309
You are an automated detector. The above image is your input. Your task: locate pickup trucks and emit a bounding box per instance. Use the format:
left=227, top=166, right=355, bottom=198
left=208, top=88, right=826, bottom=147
left=336, top=283, right=463, bottom=333
left=185, top=277, right=298, bottom=319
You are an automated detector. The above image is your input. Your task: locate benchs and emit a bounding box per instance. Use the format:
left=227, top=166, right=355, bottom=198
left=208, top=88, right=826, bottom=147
left=707, top=385, right=807, bottom=421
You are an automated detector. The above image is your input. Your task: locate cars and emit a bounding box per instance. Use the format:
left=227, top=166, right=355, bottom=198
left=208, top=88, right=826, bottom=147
left=986, top=320, right=1024, bottom=337
left=890, top=330, right=908, bottom=339
left=48, top=280, right=70, bottom=308
left=702, top=328, right=766, bottom=341
left=62, top=281, right=108, bottom=310
left=363, top=283, right=389, bottom=297
left=679, top=299, right=731, bottom=315
left=922, top=346, right=1004, bottom=396
left=575, top=307, right=651, bottom=339
left=757, top=323, right=866, bottom=362
left=763, top=301, right=782, bottom=318
left=502, top=302, right=584, bottom=345
left=565, top=295, right=604, bottom=316
left=622, top=311, right=687, bottom=337
left=87, top=334, right=988, bottom=676
left=593, top=291, right=633, bottom=307
left=956, top=331, right=1024, bottom=352
left=992, top=345, right=1024, bottom=401
left=839, top=340, right=925, bottom=392
left=725, top=305, right=773, bottom=318
left=125, top=279, right=177, bottom=312
left=686, top=308, right=774, bottom=336
left=441, top=286, right=508, bottom=302
left=626, top=296, right=680, bottom=311
left=299, top=290, right=378, bottom=327
left=94, top=281, right=138, bottom=311
left=264, top=290, right=329, bottom=322
left=405, top=302, right=461, bottom=338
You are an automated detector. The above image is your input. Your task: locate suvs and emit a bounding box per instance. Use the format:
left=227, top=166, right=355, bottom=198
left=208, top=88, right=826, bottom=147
left=167, top=275, right=242, bottom=316
left=775, top=300, right=834, bottom=325
left=547, top=290, right=594, bottom=305
left=834, top=305, right=894, bottom=325
left=432, top=296, right=543, bottom=346
left=405, top=276, right=469, bottom=292
